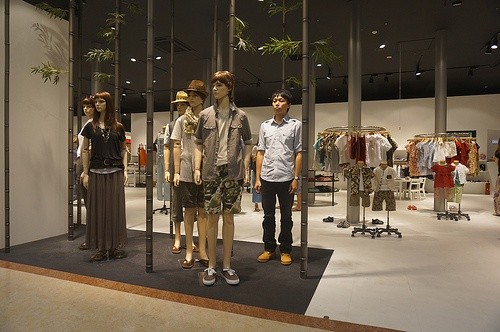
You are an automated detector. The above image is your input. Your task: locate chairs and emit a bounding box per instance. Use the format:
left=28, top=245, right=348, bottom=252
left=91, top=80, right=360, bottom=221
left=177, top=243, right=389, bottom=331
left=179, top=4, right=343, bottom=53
left=394, top=176, right=426, bottom=200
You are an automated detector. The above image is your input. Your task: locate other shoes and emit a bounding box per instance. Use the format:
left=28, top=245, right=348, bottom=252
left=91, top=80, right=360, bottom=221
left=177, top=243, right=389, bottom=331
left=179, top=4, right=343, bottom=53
left=337, top=221, right=350, bottom=228
left=407, top=204, right=417, bottom=210
left=323, top=217, right=333, bottom=222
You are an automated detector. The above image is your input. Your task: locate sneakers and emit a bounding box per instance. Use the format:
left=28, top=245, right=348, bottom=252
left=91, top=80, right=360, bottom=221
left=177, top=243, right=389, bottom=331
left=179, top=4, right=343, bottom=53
left=257, top=251, right=276, bottom=262
left=372, top=219, right=383, bottom=224
left=281, top=253, right=291, bottom=265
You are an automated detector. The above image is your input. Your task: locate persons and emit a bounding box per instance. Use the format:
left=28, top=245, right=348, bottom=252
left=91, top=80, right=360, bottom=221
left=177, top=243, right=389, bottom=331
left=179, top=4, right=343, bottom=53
left=492, top=138, right=500, bottom=216
left=77, top=96, right=96, bottom=248
left=193, top=71, right=252, bottom=284
left=81, top=92, right=127, bottom=260
left=252, top=137, right=259, bottom=172
left=254, top=90, right=303, bottom=266
left=169, top=80, right=209, bottom=269
left=163, top=91, right=199, bottom=252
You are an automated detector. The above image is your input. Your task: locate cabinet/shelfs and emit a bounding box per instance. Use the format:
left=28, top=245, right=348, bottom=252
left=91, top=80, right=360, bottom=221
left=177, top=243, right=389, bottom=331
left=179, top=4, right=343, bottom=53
left=307, top=170, right=339, bottom=206
left=127, top=163, right=157, bottom=187
left=466, top=160, right=492, bottom=183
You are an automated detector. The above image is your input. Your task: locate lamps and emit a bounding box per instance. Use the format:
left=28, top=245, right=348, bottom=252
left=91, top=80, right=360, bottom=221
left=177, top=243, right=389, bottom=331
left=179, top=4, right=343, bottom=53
left=415, top=53, right=424, bottom=76
left=368, top=75, right=374, bottom=83
left=122, top=88, right=127, bottom=97
left=326, top=64, right=332, bottom=80
left=384, top=73, right=389, bottom=82
left=480, top=31, right=500, bottom=56
left=289, top=81, right=295, bottom=90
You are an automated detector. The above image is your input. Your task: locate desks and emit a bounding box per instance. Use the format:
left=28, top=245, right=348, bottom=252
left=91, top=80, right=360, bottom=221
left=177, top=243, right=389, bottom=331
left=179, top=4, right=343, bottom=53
left=394, top=178, right=419, bottom=200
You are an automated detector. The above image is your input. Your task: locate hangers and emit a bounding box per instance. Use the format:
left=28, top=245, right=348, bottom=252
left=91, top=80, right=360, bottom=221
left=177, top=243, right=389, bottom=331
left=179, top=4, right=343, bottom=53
left=318, top=132, right=391, bottom=137
left=409, top=137, right=477, bottom=143
